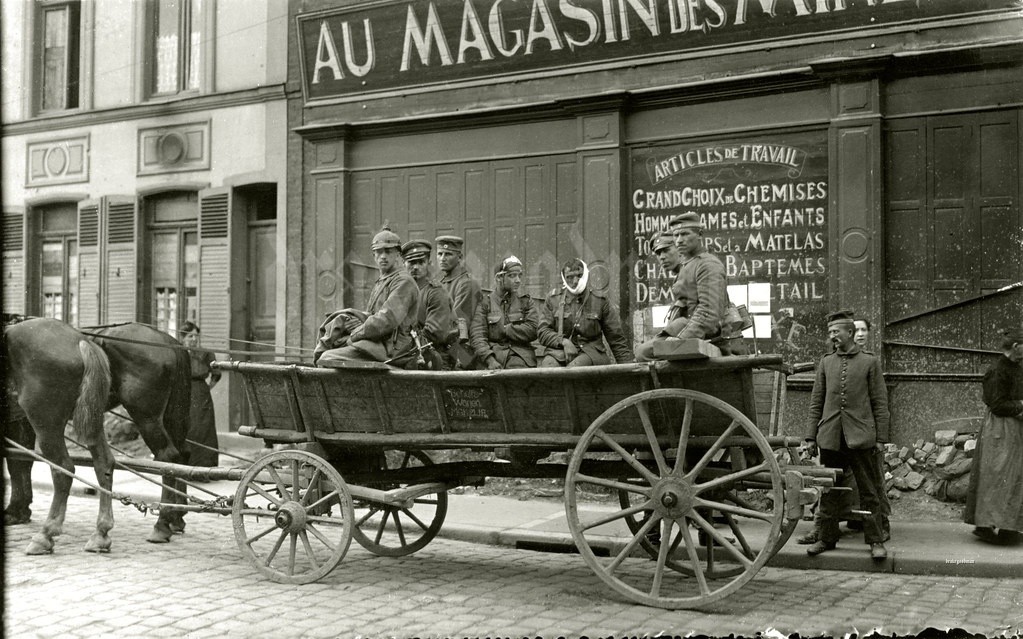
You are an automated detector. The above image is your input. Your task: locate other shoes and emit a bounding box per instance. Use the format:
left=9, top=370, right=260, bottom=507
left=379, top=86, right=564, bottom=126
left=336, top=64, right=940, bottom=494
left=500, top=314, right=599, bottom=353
left=972, top=525, right=992, bottom=543
left=808, top=540, right=837, bottom=554
left=882, top=526, right=891, bottom=542
left=998, top=529, right=1018, bottom=545
left=798, top=527, right=819, bottom=543
left=871, top=540, right=887, bottom=558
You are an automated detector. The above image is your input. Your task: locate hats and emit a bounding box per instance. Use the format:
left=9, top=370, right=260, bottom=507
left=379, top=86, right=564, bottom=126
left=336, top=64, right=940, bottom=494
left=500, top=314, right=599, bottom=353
left=372, top=230, right=401, bottom=251
left=669, top=212, right=703, bottom=231
left=401, top=239, right=432, bottom=261
left=435, top=236, right=463, bottom=252
left=494, top=251, right=522, bottom=278
left=650, top=231, right=675, bottom=253
left=825, top=310, right=854, bottom=327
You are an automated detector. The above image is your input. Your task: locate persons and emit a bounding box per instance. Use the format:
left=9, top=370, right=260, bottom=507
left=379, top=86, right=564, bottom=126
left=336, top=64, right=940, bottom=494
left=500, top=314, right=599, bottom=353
left=435, top=234, right=483, bottom=371
left=346, top=228, right=420, bottom=370
left=401, top=239, right=460, bottom=372
left=470, top=254, right=538, bottom=370
left=963, top=333, right=1023, bottom=546
left=634, top=212, right=754, bottom=363
left=153, top=320, right=222, bottom=483
left=797, top=309, right=892, bottom=564
left=537, top=256, right=635, bottom=367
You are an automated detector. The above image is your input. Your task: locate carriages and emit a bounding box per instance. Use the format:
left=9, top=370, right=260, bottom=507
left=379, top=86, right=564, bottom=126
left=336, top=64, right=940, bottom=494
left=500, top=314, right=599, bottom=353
left=2, top=314, right=842, bottom=611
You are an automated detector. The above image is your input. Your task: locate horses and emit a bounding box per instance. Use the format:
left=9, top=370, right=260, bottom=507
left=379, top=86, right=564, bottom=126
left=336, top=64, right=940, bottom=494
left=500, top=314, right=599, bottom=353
left=72, top=326, right=191, bottom=542
left=2, top=315, right=113, bottom=554
